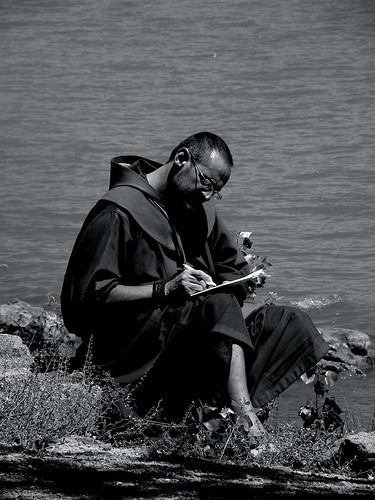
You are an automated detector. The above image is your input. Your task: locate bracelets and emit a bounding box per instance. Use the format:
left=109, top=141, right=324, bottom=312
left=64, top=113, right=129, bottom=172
left=152, top=281, right=166, bottom=300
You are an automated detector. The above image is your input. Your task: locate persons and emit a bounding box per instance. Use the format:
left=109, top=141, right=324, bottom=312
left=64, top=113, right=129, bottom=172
left=61, top=132, right=330, bottom=458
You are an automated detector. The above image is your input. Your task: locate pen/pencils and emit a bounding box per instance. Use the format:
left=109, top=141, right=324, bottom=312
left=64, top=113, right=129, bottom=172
left=183, top=264, right=216, bottom=287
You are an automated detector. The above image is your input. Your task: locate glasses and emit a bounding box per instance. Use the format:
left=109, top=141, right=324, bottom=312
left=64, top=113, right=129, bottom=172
left=192, top=158, right=223, bottom=200
